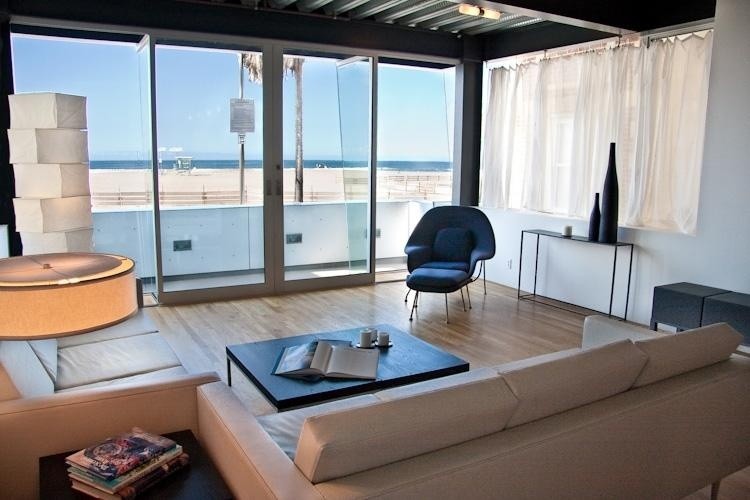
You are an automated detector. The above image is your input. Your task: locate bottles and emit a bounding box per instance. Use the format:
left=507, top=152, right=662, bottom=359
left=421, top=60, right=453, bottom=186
left=588, top=192, right=602, bottom=241
left=600, top=142, right=619, bottom=244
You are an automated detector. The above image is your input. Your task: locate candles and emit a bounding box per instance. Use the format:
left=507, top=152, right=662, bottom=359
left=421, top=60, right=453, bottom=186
left=562, top=225, right=572, bottom=235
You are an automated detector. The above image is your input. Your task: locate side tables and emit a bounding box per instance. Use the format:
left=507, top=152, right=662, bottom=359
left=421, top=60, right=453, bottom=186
left=39, top=429, right=235, bottom=499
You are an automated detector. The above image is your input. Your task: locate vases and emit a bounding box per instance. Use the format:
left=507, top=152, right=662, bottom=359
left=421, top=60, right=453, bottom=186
left=589, top=142, right=621, bottom=240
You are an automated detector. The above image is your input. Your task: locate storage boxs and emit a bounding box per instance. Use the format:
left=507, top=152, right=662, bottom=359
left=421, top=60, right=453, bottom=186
left=6, top=93, right=96, bottom=255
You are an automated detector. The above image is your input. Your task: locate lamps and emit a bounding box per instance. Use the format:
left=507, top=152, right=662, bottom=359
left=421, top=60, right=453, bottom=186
left=0, top=252, right=139, bottom=342
left=460, top=4, right=503, bottom=20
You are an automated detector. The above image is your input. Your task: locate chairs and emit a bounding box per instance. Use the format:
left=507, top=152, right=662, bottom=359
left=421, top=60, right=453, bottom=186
left=403, top=205, right=495, bottom=324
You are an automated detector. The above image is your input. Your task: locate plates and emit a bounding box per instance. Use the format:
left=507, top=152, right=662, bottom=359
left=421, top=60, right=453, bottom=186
left=356, top=342, right=376, bottom=349
left=375, top=340, right=394, bottom=349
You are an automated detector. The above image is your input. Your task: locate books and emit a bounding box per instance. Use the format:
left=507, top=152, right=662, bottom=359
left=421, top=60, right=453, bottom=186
left=272, top=339, right=380, bottom=384
left=63, top=425, right=191, bottom=500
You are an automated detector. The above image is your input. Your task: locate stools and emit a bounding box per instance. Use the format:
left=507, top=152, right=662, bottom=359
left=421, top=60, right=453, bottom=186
left=649, top=282, right=731, bottom=332
left=701, top=291, right=750, bottom=346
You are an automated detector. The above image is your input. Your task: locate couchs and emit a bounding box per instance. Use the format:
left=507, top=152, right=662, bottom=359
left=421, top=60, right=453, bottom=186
left=0, top=275, right=220, bottom=500
left=198, top=314, right=749, bottom=500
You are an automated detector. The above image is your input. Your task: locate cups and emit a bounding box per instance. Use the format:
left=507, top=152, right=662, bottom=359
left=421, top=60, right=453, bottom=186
left=360, top=330, right=372, bottom=347
left=370, top=328, right=377, bottom=341
left=378, top=332, right=390, bottom=346
left=562, top=226, right=573, bottom=237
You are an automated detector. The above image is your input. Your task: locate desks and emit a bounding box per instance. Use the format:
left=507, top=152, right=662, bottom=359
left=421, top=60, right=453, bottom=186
left=517, top=228, right=634, bottom=320
